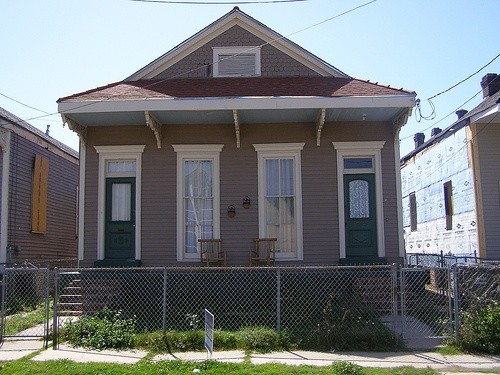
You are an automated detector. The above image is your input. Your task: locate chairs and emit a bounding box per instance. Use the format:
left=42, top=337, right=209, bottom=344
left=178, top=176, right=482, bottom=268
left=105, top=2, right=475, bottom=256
left=198, top=238, right=228, bottom=273
left=249, top=237, right=280, bottom=273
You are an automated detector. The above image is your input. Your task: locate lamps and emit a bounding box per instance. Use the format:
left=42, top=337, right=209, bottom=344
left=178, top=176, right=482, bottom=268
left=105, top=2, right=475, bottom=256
left=243, top=195, right=251, bottom=209
left=227, top=204, right=236, bottom=218
